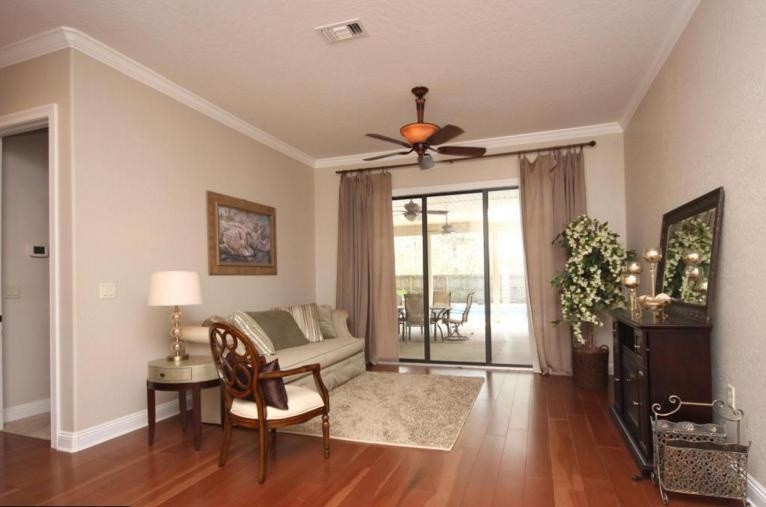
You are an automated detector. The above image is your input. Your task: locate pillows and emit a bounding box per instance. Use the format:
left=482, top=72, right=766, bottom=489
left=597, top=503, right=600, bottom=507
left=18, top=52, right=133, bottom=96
left=228, top=303, right=336, bottom=354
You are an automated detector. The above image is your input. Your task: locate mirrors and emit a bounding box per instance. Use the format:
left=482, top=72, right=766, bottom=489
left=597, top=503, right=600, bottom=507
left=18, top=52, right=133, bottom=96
left=654, top=186, right=726, bottom=327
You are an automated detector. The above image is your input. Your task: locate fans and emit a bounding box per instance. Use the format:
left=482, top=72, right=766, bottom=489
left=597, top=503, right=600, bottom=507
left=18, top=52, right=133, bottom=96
left=362, top=86, right=487, bottom=169
left=393, top=198, right=449, bottom=221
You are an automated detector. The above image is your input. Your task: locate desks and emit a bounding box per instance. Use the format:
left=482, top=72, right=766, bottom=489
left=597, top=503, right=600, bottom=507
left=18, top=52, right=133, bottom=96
left=397, top=306, right=450, bottom=345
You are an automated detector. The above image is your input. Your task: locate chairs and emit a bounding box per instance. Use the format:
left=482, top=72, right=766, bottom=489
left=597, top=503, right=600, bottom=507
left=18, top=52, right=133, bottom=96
left=388, top=290, right=476, bottom=345
left=208, top=322, right=330, bottom=484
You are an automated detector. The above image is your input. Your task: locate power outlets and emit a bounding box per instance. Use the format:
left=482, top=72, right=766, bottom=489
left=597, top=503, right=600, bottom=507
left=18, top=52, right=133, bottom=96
left=726, top=384, right=735, bottom=408
left=100, top=283, right=116, bottom=299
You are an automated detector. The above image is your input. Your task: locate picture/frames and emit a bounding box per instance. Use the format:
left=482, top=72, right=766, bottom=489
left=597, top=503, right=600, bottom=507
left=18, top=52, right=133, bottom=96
left=207, top=191, right=278, bottom=275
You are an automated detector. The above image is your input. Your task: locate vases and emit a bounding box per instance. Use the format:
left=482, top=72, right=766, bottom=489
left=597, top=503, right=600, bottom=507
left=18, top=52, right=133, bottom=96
left=573, top=345, right=610, bottom=390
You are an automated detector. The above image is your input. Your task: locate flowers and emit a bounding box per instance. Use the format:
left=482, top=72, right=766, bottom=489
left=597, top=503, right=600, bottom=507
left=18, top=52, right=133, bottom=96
left=662, top=207, right=716, bottom=306
left=550, top=214, right=631, bottom=348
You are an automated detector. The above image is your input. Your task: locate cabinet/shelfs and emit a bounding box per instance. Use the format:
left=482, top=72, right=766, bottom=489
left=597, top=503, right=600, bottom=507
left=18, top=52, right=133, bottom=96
left=608, top=308, right=713, bottom=481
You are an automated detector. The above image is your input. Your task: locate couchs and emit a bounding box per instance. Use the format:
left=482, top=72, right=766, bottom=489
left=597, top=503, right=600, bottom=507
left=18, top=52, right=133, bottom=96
left=181, top=306, right=366, bottom=423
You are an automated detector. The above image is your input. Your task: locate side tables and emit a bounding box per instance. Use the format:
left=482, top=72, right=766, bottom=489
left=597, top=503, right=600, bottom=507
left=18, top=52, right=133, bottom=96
left=147, top=359, right=226, bottom=451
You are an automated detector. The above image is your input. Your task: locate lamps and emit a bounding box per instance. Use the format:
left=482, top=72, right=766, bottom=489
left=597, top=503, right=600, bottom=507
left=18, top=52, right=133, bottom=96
left=147, top=272, right=202, bottom=364
left=400, top=86, right=442, bottom=143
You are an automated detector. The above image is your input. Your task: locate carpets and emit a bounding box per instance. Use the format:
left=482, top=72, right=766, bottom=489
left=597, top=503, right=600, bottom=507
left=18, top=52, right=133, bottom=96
left=268, top=375, right=485, bottom=451
